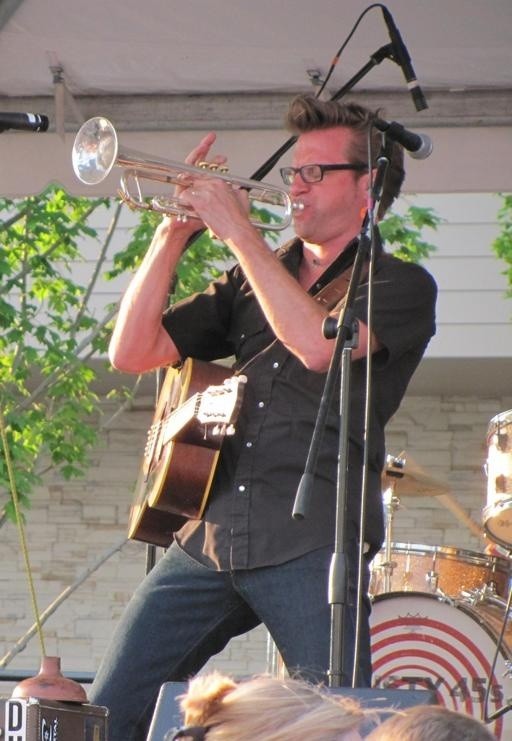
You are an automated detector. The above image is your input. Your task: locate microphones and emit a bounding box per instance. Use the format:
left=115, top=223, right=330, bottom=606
left=0, top=105, right=50, bottom=136
left=369, top=112, right=435, bottom=166
left=384, top=7, right=430, bottom=115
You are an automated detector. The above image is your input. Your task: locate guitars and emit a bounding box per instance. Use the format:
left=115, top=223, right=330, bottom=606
left=124, top=358, right=248, bottom=551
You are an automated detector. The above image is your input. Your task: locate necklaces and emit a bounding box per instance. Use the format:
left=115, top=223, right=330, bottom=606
left=300, top=248, right=344, bottom=268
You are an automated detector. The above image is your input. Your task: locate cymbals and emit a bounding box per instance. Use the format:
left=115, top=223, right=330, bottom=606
left=380, top=464, right=454, bottom=497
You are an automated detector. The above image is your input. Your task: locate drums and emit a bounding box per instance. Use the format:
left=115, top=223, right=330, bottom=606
left=368, top=542, right=512, bottom=599
left=480, top=410, right=512, bottom=551
left=368, top=591, right=511, bottom=741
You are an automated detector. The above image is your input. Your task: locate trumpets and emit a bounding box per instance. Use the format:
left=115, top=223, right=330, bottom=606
left=70, top=117, right=305, bottom=231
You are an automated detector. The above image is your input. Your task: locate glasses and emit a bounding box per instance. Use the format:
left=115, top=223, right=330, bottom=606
left=279, top=163, right=365, bottom=184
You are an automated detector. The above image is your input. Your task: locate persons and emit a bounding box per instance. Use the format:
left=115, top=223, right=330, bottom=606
left=177, top=671, right=364, bottom=741
left=361, top=705, right=495, bottom=741
left=88, top=96, right=437, bottom=735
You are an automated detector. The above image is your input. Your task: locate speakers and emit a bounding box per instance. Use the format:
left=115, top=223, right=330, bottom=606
left=143, top=679, right=437, bottom=740
